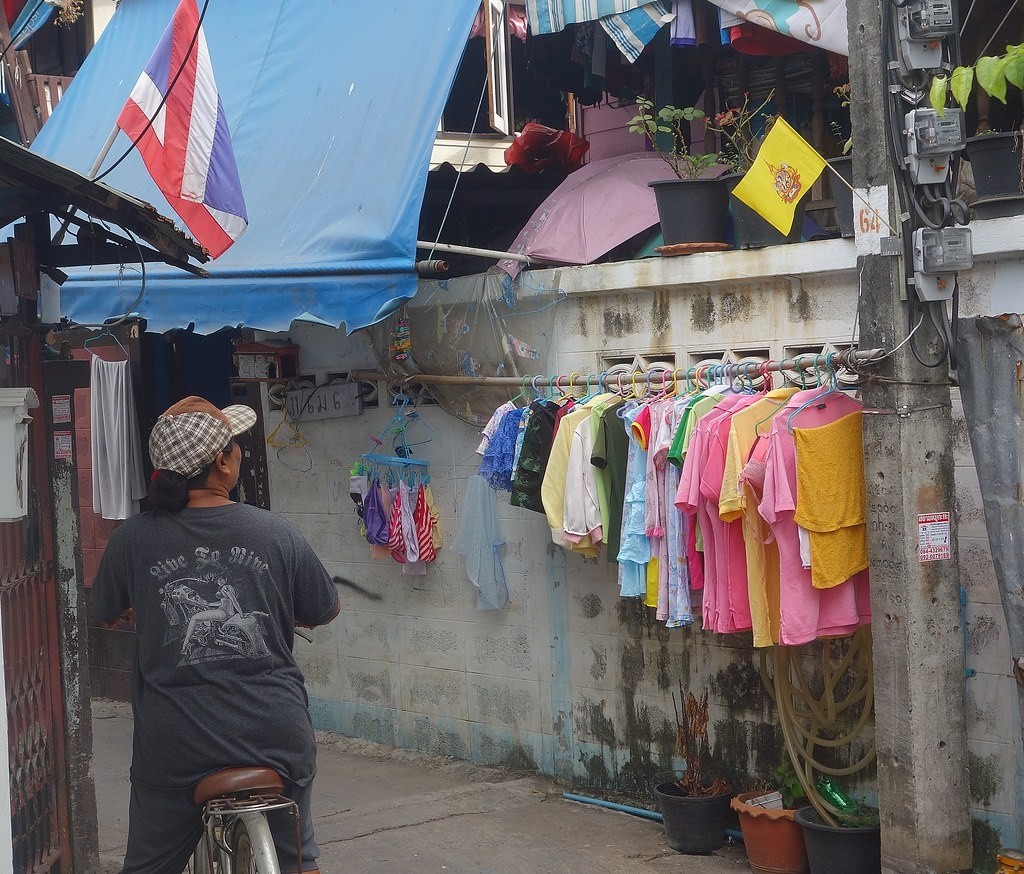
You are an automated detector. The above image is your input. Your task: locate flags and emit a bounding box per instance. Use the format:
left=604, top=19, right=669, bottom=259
left=118, top=0, right=249, bottom=262
left=731, top=118, right=826, bottom=238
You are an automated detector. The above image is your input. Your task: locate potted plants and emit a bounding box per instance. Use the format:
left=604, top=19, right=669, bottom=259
left=654, top=675, right=880, bottom=874
left=632, top=97, right=728, bottom=244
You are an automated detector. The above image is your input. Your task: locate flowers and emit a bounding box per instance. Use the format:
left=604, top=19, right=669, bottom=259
left=704, top=89, right=783, bottom=171
left=830, top=84, right=854, bottom=156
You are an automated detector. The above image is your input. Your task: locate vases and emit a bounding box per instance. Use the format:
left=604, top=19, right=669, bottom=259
left=824, top=158, right=855, bottom=238
left=719, top=174, right=803, bottom=246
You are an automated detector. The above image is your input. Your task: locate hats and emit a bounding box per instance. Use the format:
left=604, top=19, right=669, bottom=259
left=148, top=395, right=257, bottom=480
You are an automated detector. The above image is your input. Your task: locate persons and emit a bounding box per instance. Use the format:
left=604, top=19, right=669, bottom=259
left=90, top=396, right=340, bottom=873
left=959, top=0, right=1024, bottom=136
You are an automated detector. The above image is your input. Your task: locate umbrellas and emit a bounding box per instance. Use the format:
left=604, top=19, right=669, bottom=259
left=497, top=150, right=732, bottom=280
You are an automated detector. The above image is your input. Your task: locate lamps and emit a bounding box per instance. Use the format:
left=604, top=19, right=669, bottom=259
left=36, top=264, right=67, bottom=285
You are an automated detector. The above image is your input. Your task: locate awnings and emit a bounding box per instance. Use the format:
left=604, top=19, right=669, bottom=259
left=1, top=0, right=545, bottom=338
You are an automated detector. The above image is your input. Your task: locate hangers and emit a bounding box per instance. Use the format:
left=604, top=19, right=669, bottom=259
left=267, top=404, right=306, bottom=448
left=277, top=419, right=312, bottom=473
left=392, top=399, right=434, bottom=448
left=84, top=321, right=128, bottom=363
left=510, top=351, right=846, bottom=441
left=487, top=255, right=568, bottom=322
left=446, top=425, right=488, bottom=481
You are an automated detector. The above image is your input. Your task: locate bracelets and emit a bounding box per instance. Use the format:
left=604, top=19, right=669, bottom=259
left=978, top=118, right=991, bottom=123
